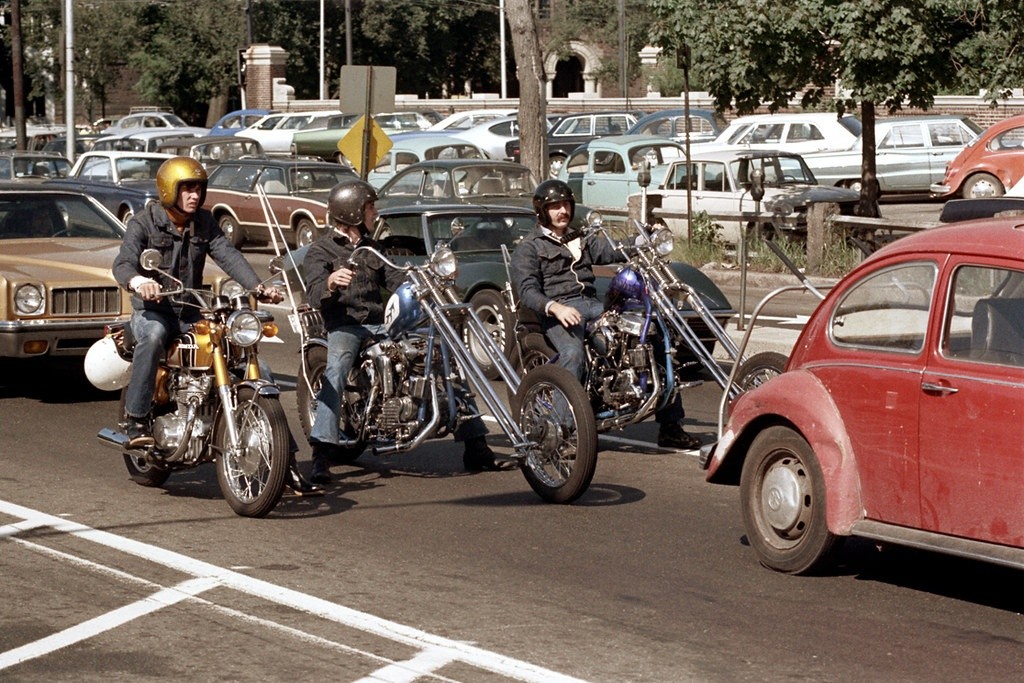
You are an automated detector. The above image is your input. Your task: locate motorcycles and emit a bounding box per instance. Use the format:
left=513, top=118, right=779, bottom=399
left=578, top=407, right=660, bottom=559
left=83, top=184, right=790, bottom=523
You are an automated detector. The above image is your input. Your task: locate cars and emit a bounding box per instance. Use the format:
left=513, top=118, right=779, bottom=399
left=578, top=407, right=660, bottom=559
left=0, top=184, right=136, bottom=359
left=698, top=195, right=1024, bottom=577
left=929, top=113, right=1024, bottom=199
left=40, top=150, right=177, bottom=228
left=199, top=158, right=361, bottom=248
left=365, top=158, right=540, bottom=241
left=0, top=107, right=985, bottom=207
left=626, top=150, right=862, bottom=249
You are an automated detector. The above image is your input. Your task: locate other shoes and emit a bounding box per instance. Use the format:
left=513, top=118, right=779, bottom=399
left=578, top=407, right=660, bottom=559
left=127, top=415, right=155, bottom=446
left=463, top=446, right=518, bottom=470
left=285, top=461, right=326, bottom=498
left=558, top=446, right=577, bottom=461
left=312, top=443, right=333, bottom=482
left=657, top=424, right=702, bottom=449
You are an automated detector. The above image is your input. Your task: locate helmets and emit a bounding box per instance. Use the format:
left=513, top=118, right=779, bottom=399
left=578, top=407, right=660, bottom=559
left=83, top=335, right=132, bottom=391
left=156, top=156, right=210, bottom=209
left=328, top=180, right=379, bottom=225
left=532, top=179, right=576, bottom=227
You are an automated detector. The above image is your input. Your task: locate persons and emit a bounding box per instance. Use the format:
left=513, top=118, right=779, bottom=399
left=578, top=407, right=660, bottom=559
left=304, top=179, right=518, bottom=485
left=510, top=181, right=704, bottom=449
left=113, top=157, right=327, bottom=497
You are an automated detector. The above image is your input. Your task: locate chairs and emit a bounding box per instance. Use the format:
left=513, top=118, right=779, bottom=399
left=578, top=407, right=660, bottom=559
left=478, top=179, right=504, bottom=194
left=35, top=165, right=50, bottom=175
left=15, top=165, right=29, bottom=176
left=969, top=297, right=1024, bottom=368
left=313, top=176, right=339, bottom=190
left=0, top=201, right=56, bottom=239
left=258, top=175, right=280, bottom=187
left=433, top=180, right=459, bottom=196
left=681, top=175, right=699, bottom=190
